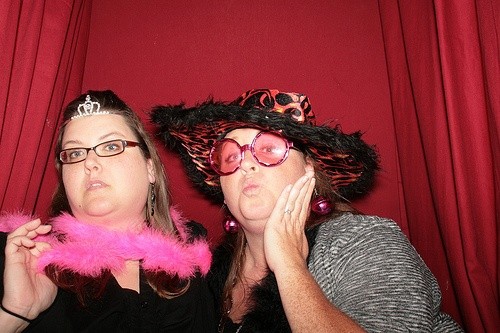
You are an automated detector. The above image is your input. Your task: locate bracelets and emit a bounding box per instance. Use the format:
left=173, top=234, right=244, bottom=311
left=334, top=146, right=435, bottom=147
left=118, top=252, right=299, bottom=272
left=0, top=300, right=35, bottom=323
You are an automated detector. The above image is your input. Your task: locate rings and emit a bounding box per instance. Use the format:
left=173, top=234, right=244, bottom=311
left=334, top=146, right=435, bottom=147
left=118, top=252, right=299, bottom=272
left=284, top=208, right=292, bottom=214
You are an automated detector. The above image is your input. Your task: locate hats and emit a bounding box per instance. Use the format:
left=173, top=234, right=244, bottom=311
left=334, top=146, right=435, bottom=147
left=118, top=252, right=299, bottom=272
left=148, top=89, right=378, bottom=202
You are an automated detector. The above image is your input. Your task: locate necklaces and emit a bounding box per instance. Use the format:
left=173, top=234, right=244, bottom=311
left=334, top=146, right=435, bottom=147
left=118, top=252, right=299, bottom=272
left=217, top=238, right=247, bottom=333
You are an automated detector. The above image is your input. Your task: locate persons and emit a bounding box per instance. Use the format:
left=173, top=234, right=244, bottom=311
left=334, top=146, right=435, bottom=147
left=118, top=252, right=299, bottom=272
left=0, top=89, right=220, bottom=333
left=149, top=86, right=465, bottom=333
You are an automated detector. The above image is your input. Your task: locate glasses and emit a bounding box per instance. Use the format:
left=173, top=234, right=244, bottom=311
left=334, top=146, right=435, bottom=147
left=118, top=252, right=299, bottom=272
left=203, top=130, right=294, bottom=176
left=56, top=139, right=139, bottom=165
